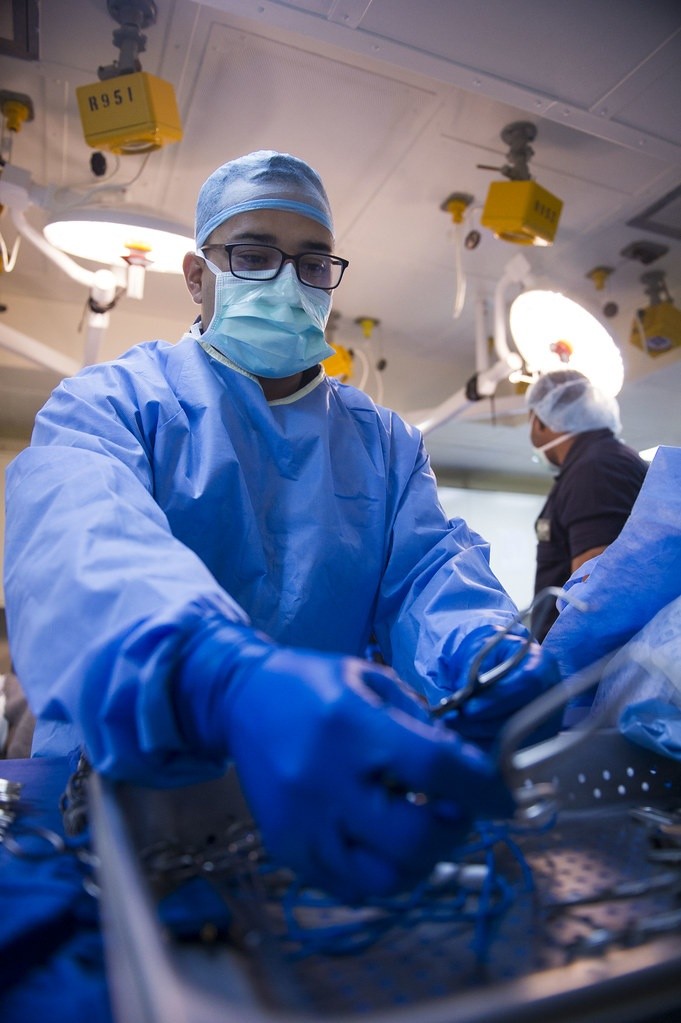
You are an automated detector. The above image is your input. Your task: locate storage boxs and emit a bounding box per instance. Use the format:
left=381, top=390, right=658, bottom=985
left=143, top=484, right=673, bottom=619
left=87, top=728, right=681, bottom=1023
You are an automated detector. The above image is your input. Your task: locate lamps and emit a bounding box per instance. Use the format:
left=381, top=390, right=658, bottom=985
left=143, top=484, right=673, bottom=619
left=412, top=286, right=626, bottom=452
left=77, top=0, right=180, bottom=158
left=629, top=271, right=681, bottom=359
left=0, top=163, right=198, bottom=276
left=480, top=122, right=565, bottom=248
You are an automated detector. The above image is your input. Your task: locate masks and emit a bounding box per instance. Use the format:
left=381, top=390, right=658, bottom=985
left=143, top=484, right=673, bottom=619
left=531, top=445, right=561, bottom=476
left=197, top=251, right=336, bottom=379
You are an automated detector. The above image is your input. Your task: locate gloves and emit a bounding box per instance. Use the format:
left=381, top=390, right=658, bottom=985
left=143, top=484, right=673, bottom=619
left=180, top=622, right=498, bottom=905
left=443, top=624, right=564, bottom=749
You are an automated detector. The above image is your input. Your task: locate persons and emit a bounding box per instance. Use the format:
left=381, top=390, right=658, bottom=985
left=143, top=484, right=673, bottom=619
left=523, top=367, right=651, bottom=645
left=6, top=149, right=545, bottom=904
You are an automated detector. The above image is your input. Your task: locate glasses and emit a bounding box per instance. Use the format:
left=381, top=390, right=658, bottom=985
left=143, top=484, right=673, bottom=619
left=199, top=242, right=348, bottom=289
